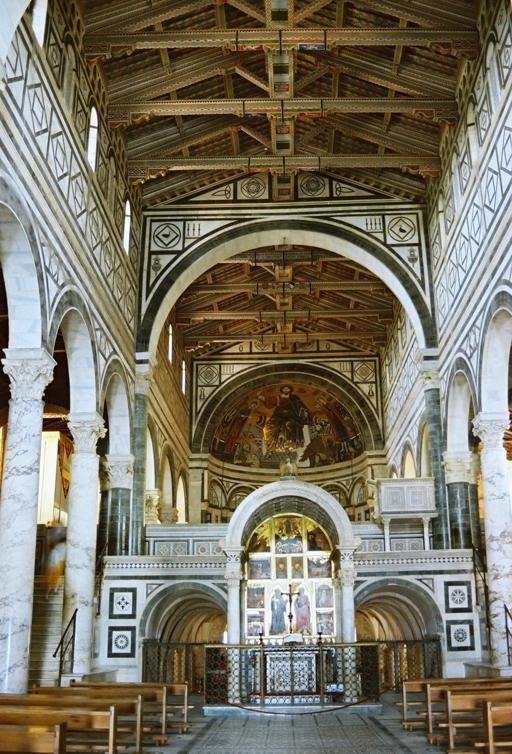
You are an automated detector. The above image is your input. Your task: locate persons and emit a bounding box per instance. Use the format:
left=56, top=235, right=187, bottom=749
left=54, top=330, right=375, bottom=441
left=270, top=587, right=288, bottom=634
left=264, top=384, right=313, bottom=448
left=295, top=586, right=311, bottom=631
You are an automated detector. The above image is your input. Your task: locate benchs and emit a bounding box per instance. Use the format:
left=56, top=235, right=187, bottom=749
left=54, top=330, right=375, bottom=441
left=1, top=678, right=196, bottom=754
left=391, top=678, right=511, bottom=754
